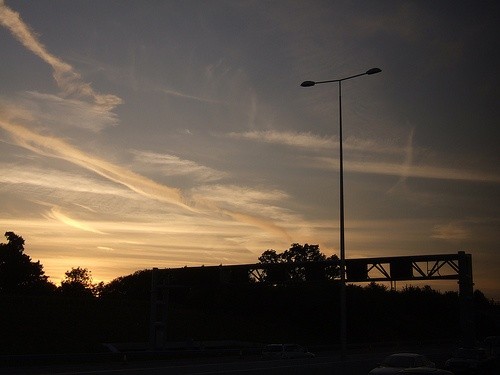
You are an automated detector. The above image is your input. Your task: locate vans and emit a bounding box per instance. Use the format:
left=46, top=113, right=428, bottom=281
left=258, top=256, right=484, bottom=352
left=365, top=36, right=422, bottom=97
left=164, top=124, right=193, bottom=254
left=262, top=344, right=315, bottom=361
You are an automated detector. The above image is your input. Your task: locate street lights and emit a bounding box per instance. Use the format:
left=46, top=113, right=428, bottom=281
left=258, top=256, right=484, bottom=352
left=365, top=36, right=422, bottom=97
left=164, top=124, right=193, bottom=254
left=300, top=68, right=383, bottom=361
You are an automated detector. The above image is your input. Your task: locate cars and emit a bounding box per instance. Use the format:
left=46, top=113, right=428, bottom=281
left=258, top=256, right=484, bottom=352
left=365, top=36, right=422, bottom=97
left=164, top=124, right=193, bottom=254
left=402, top=335, right=500, bottom=374
left=368, top=352, right=433, bottom=375
